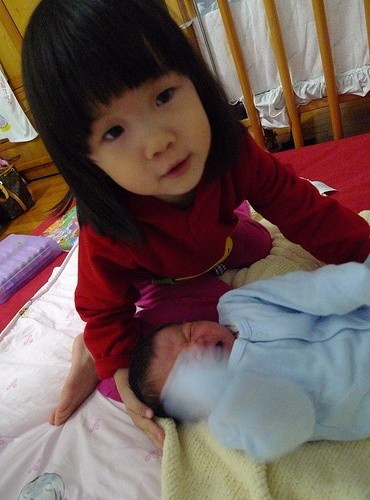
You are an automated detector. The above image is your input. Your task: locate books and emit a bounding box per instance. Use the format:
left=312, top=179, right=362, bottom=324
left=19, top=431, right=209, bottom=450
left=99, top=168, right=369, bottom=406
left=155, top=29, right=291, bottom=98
left=39, top=204, right=80, bottom=252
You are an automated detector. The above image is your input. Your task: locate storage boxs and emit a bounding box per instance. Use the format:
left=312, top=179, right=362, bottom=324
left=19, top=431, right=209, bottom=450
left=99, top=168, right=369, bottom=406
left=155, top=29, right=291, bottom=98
left=0, top=233, right=62, bottom=306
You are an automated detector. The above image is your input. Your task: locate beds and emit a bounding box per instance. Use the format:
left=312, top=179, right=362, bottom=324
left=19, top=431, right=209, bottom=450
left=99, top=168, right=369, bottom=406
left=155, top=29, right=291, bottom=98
left=0, top=133, right=370, bottom=500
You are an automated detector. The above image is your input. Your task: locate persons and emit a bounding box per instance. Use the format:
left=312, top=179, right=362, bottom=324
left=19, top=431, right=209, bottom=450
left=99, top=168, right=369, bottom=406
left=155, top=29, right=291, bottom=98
left=128, top=262, right=370, bottom=461
left=21, top=0, right=370, bottom=454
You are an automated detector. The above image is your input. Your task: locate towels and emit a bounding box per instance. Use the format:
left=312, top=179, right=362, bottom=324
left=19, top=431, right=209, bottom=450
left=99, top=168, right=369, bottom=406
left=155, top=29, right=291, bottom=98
left=141, top=207, right=370, bottom=500
